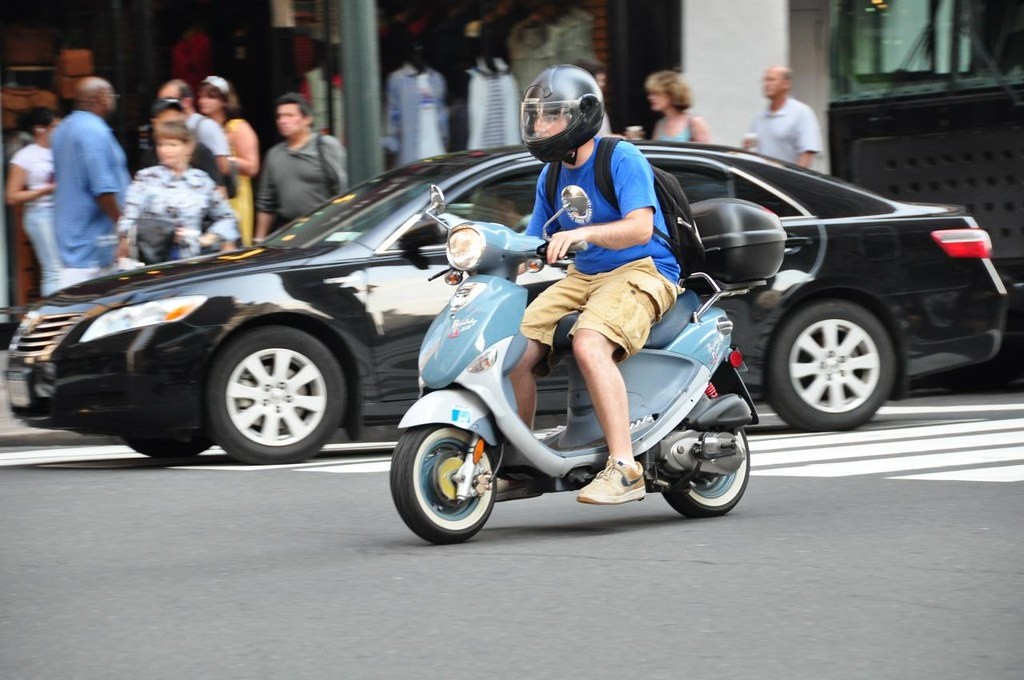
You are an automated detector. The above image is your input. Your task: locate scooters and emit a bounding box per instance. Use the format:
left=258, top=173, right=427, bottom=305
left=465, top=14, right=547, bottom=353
left=389, top=183, right=789, bottom=546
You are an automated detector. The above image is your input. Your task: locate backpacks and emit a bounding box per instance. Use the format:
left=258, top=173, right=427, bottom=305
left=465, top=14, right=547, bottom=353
left=546, top=137, right=704, bottom=279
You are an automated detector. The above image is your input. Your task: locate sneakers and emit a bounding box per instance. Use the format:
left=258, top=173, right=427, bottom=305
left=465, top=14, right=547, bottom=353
left=577, top=458, right=646, bottom=504
left=489, top=479, right=542, bottom=501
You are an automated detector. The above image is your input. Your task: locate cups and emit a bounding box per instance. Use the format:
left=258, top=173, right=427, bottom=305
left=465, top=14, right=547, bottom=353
left=744, top=133, right=759, bottom=153
left=625, top=126, right=644, bottom=141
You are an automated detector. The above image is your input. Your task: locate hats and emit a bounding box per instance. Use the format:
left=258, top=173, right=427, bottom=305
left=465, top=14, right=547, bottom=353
left=151, top=98, right=185, bottom=120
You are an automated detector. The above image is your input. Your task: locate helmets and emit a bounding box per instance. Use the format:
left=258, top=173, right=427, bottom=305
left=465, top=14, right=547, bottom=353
left=523, top=63, right=604, bottom=163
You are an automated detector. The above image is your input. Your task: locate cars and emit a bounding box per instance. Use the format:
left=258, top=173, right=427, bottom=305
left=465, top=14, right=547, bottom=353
left=2, top=138, right=1014, bottom=466
left=822, top=1, right=1024, bottom=297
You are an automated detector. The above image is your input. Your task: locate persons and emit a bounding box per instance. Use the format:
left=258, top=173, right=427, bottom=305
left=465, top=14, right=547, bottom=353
left=466, top=53, right=523, bottom=151
left=382, top=55, right=449, bottom=166
left=743, top=66, right=828, bottom=176
left=509, top=64, right=681, bottom=506
left=624, top=70, right=710, bottom=142
left=5, top=74, right=260, bottom=300
left=253, top=92, right=348, bottom=248
